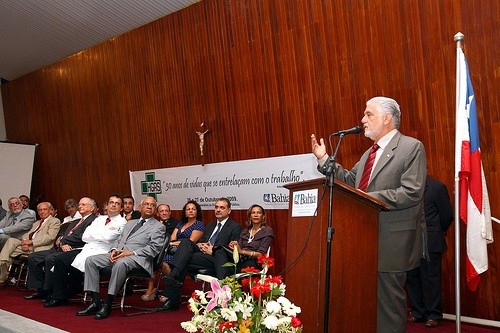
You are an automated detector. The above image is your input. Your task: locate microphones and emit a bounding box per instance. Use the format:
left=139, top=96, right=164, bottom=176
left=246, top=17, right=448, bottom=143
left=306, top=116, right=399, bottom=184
left=332, top=124, right=363, bottom=136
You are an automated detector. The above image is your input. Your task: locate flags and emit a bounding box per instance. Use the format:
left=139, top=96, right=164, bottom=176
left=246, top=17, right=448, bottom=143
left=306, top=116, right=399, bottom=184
left=459, top=49, right=493, bottom=292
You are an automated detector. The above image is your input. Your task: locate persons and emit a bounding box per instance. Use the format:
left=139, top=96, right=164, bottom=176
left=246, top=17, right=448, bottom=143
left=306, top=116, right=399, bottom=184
left=407, top=174, right=453, bottom=327
left=102, top=201, right=108, bottom=215
left=51, top=204, right=63, bottom=224
left=19, top=195, right=36, bottom=221
left=94, top=200, right=101, bottom=216
left=310, top=97, right=428, bottom=333
left=0, top=199, right=7, bottom=221
left=156, top=204, right=180, bottom=233
left=120, top=195, right=140, bottom=220
left=0, top=198, right=35, bottom=252
left=214, top=203, right=273, bottom=281
left=23, top=197, right=97, bottom=302
left=0, top=202, right=61, bottom=289
left=76, top=196, right=166, bottom=320
left=140, top=201, right=207, bottom=302
left=61, top=199, right=82, bottom=225
left=44, top=194, right=127, bottom=307
left=152, top=198, right=243, bottom=312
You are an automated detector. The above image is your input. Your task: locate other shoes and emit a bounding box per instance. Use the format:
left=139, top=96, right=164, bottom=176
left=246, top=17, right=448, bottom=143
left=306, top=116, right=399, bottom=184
left=141, top=292, right=155, bottom=302
left=116, top=288, right=135, bottom=297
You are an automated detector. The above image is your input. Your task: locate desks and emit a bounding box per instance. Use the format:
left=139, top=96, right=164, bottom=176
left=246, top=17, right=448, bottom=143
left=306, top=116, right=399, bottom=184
left=281, top=176, right=391, bottom=333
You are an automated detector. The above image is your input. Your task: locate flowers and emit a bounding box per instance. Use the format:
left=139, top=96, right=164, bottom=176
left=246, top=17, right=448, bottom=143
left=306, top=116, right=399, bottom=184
left=180, top=244, right=303, bottom=333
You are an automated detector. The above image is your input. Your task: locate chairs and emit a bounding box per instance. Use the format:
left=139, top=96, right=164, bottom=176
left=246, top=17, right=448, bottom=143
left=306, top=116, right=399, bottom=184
left=4, top=233, right=271, bottom=316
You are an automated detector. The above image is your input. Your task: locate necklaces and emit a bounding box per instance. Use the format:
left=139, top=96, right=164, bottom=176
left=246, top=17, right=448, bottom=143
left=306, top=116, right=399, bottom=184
left=247, top=226, right=261, bottom=244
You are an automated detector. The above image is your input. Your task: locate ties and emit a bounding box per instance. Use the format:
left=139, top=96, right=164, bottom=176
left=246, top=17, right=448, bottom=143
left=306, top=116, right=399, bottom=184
left=357, top=144, right=380, bottom=193
left=210, top=223, right=222, bottom=246
left=122, top=220, right=146, bottom=250
left=68, top=218, right=84, bottom=235
left=29, top=220, right=44, bottom=240
left=105, top=218, right=111, bottom=225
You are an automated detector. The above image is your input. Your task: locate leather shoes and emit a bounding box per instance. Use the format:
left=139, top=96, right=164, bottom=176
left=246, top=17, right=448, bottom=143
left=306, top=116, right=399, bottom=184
left=43, top=297, right=52, bottom=302
left=43, top=299, right=68, bottom=306
left=24, top=293, right=46, bottom=298
left=95, top=304, right=112, bottom=319
left=426, top=320, right=439, bottom=327
left=154, top=301, right=182, bottom=312
left=163, top=273, right=184, bottom=287
left=76, top=300, right=101, bottom=315
left=407, top=316, right=421, bottom=323
